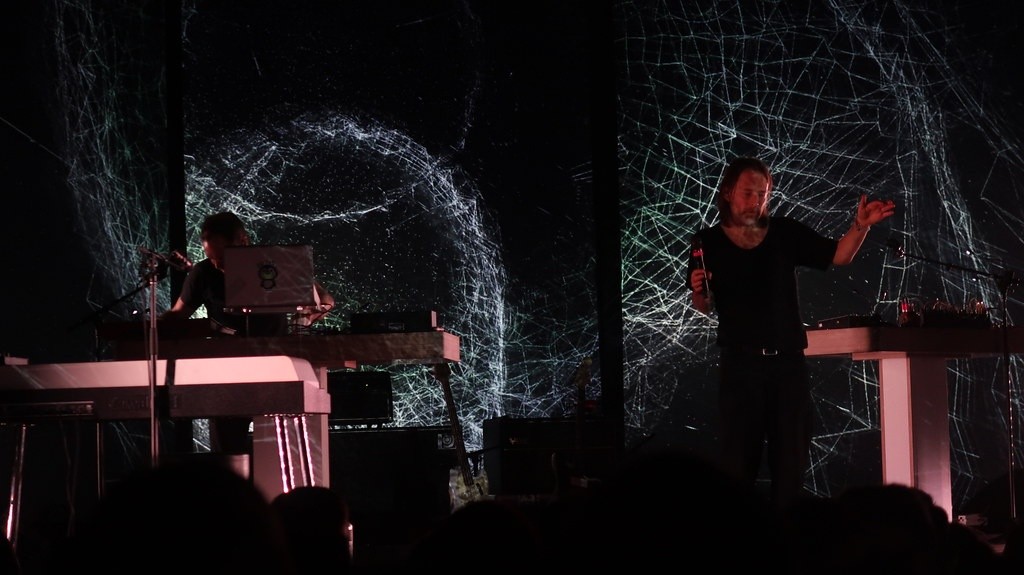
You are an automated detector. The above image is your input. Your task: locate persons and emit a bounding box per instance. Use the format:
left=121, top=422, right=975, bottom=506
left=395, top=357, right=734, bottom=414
left=686, top=156, right=896, bottom=497
left=62, top=453, right=1000, bottom=575
left=161, top=212, right=335, bottom=483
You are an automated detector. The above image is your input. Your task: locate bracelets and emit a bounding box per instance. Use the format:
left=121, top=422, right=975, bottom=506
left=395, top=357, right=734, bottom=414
left=854, top=219, right=870, bottom=232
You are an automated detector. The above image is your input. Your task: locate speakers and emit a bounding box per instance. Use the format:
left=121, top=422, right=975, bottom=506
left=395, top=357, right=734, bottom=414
left=325, top=371, right=456, bottom=517
left=483, top=419, right=604, bottom=499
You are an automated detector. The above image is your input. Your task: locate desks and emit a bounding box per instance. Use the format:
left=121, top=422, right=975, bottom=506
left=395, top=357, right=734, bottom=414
left=112, top=332, right=460, bottom=502
left=0, top=380, right=330, bottom=546
left=803, top=325, right=1024, bottom=522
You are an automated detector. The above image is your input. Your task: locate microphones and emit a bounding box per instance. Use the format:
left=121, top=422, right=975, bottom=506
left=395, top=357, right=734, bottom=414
left=173, top=251, right=191, bottom=270
left=691, top=238, right=710, bottom=300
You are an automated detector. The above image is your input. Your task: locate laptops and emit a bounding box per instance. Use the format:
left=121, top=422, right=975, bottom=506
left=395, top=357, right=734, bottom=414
left=223, top=245, right=316, bottom=307
